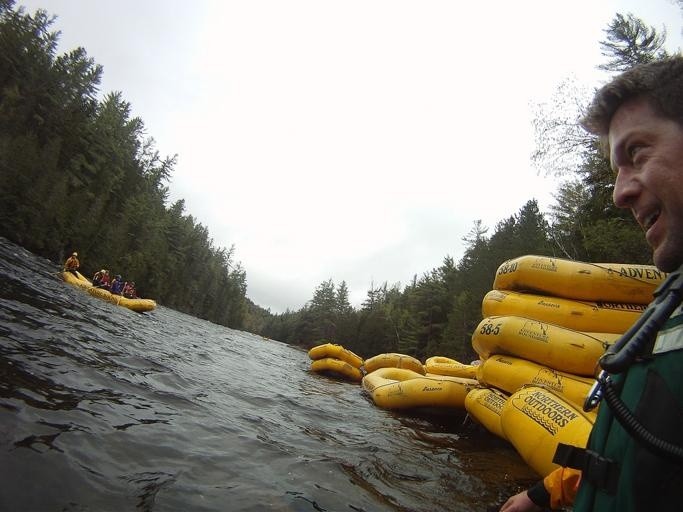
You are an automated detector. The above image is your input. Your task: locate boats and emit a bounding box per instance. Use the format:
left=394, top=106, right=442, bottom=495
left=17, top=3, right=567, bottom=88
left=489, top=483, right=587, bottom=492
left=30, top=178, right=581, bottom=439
left=62, top=271, right=157, bottom=310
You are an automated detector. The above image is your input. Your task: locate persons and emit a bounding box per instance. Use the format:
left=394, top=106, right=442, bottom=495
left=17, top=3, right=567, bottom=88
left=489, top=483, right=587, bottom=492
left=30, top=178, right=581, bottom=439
left=64, top=252, right=80, bottom=280
left=498, top=51, right=683, bottom=511
left=92, top=269, right=138, bottom=299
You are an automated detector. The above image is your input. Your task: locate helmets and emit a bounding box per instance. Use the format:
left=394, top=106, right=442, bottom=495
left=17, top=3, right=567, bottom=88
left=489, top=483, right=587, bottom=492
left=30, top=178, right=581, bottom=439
left=73, top=252, right=77, bottom=256
left=100, top=269, right=136, bottom=288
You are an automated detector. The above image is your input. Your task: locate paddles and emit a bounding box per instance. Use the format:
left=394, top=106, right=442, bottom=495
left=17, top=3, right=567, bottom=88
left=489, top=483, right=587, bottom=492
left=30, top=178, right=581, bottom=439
left=87, top=284, right=107, bottom=293
left=116, top=284, right=126, bottom=306
